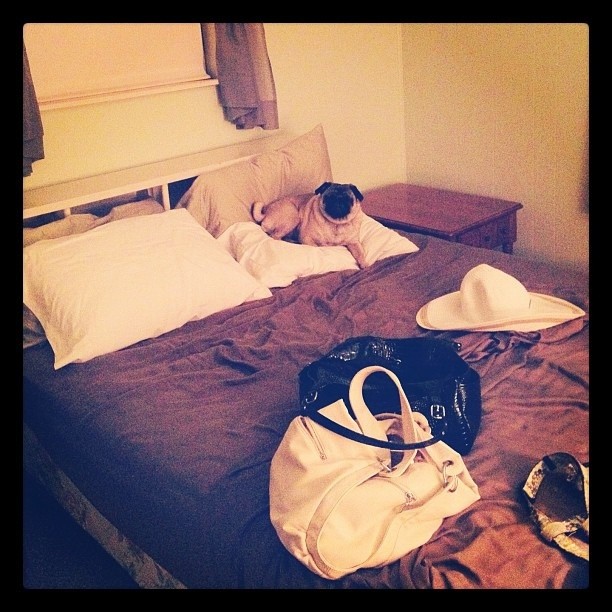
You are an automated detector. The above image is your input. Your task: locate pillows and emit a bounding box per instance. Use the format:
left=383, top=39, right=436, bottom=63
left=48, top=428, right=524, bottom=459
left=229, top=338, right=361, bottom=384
left=23, top=207, right=273, bottom=370
left=216, top=209, right=421, bottom=294
left=23, top=196, right=165, bottom=355
left=177, top=125, right=335, bottom=233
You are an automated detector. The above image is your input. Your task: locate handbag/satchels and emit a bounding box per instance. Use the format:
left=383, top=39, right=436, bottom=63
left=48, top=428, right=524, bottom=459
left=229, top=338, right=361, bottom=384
left=268, top=364, right=483, bottom=582
left=298, top=335, right=484, bottom=457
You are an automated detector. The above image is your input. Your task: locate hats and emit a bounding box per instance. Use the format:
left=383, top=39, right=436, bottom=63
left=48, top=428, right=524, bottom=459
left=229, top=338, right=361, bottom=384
left=415, top=263, right=586, bottom=333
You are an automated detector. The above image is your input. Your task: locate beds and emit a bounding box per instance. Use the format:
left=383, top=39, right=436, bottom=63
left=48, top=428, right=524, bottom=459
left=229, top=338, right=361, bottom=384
left=23, top=133, right=589, bottom=593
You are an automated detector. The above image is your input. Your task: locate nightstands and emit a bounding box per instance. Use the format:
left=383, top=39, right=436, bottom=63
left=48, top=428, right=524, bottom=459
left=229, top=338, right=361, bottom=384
left=359, top=183, right=524, bottom=257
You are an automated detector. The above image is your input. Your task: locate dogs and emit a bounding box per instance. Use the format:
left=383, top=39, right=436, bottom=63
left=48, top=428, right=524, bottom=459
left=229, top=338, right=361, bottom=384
left=252, top=180, right=370, bottom=270
